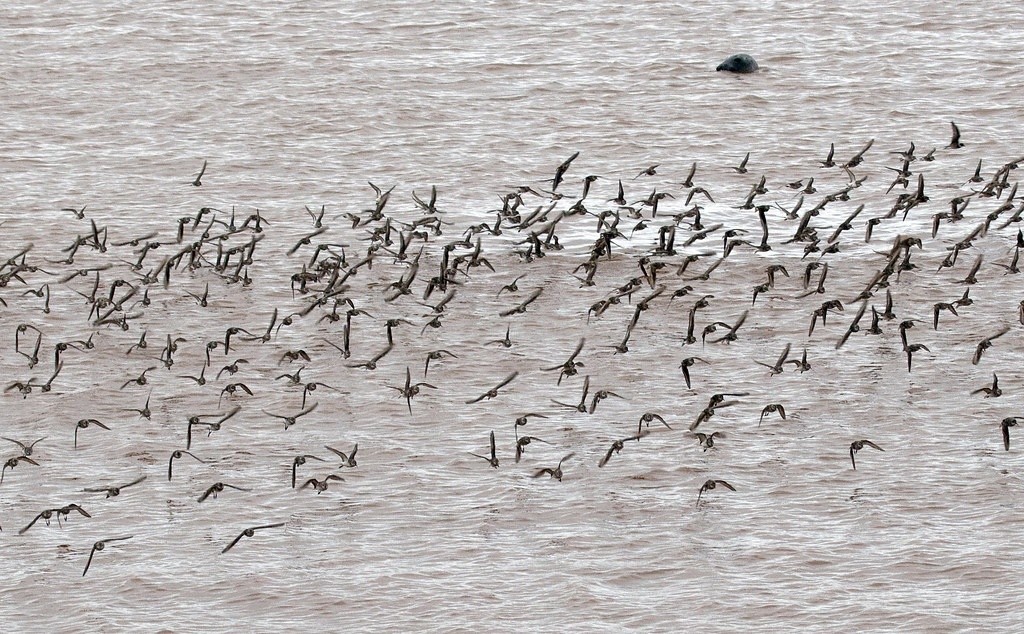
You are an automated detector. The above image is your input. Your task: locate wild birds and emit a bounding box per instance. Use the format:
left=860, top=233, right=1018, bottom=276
left=0, top=122, right=1024, bottom=577
